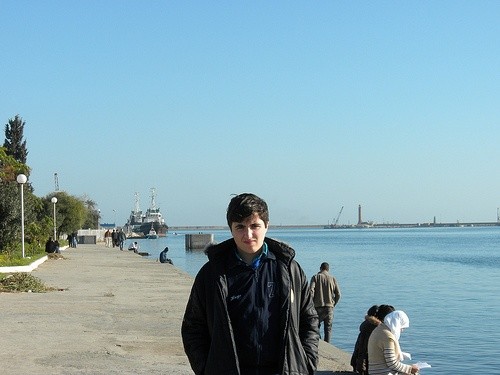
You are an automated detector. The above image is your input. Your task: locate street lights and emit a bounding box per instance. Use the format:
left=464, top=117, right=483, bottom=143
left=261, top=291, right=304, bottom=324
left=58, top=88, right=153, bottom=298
left=50, top=196, right=58, bottom=242
left=17, top=174, right=28, bottom=258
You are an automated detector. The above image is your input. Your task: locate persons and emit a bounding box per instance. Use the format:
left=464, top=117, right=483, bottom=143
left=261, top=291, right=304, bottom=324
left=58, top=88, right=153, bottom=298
left=310, top=262, right=341, bottom=344
left=67, top=231, right=78, bottom=248
left=160, top=247, right=173, bottom=265
left=105, top=229, right=111, bottom=248
left=181, top=193, right=320, bottom=375
left=45, top=237, right=61, bottom=254
left=114, top=229, right=119, bottom=247
left=118, top=229, right=126, bottom=251
left=368, top=310, right=419, bottom=375
left=128, top=241, right=137, bottom=253
left=350, top=305, right=379, bottom=373
left=112, top=229, right=116, bottom=248
left=356, top=305, right=396, bottom=375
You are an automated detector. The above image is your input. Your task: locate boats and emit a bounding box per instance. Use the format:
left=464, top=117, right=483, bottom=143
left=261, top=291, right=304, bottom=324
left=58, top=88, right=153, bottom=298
left=140, top=187, right=169, bottom=239
left=120, top=191, right=145, bottom=239
